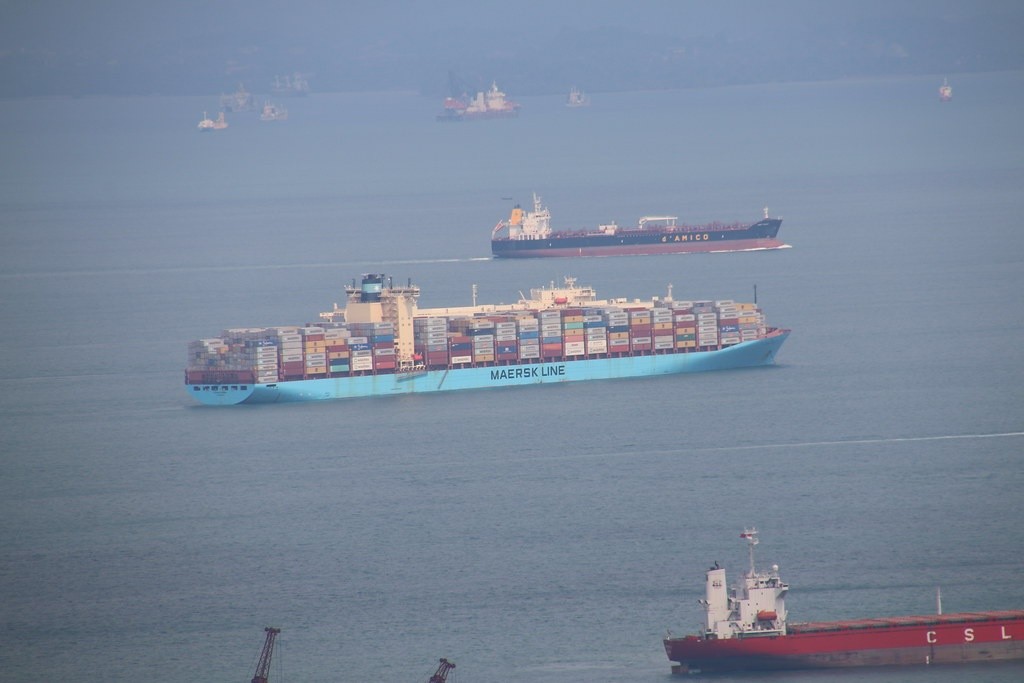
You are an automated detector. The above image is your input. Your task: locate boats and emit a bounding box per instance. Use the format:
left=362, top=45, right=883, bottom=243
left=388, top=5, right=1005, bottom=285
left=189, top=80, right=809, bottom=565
left=937, top=78, right=953, bottom=102
left=663, top=524, right=1022, bottom=671
left=557, top=88, right=590, bottom=107
left=195, top=69, right=311, bottom=132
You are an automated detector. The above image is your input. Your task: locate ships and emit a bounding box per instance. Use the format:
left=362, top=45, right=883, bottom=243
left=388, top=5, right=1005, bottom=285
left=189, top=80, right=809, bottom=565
left=491, top=192, right=784, bottom=259
left=182, top=271, right=795, bottom=408
left=433, top=80, right=522, bottom=120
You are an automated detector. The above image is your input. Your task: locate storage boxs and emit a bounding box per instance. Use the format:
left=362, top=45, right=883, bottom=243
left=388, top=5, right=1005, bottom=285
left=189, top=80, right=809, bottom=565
left=186, top=297, right=766, bottom=385
